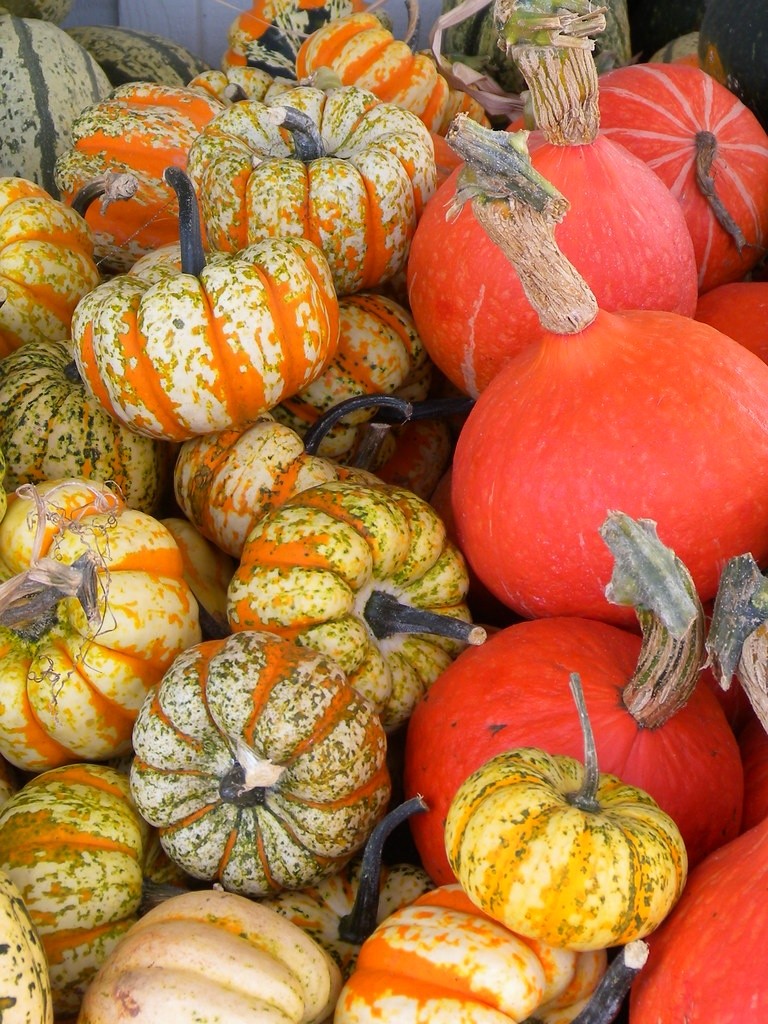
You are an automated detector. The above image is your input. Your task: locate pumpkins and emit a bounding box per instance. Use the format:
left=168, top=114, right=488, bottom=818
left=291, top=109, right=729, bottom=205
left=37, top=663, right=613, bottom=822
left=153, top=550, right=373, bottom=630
left=0, top=0, right=768, bottom=1024
